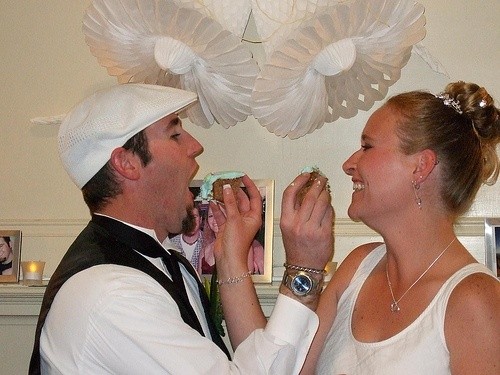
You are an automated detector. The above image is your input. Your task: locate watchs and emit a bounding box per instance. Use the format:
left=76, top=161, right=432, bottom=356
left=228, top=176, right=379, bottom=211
left=282, top=270, right=323, bottom=296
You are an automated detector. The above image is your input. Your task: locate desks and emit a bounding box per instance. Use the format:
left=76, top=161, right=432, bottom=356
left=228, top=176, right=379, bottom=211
left=0, top=278, right=328, bottom=375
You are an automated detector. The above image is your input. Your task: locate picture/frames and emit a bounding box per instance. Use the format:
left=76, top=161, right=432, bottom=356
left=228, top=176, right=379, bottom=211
left=0, top=230, right=22, bottom=282
left=168, top=180, right=275, bottom=284
left=484, top=217, right=500, bottom=279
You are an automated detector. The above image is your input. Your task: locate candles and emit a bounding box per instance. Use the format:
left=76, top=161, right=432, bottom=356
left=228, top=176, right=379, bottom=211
left=24, top=261, right=42, bottom=279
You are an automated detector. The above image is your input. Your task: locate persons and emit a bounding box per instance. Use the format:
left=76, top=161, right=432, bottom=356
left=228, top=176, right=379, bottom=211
left=169, top=205, right=264, bottom=275
left=207, top=81, right=500, bottom=375
left=29, top=81, right=333, bottom=375
left=0, top=236, right=14, bottom=275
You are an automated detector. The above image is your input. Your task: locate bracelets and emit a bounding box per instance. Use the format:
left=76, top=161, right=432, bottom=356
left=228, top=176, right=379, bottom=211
left=215, top=271, right=252, bottom=287
left=284, top=263, right=327, bottom=274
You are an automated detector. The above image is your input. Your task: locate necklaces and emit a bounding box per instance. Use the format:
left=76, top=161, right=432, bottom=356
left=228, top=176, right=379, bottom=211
left=386, top=237, right=457, bottom=312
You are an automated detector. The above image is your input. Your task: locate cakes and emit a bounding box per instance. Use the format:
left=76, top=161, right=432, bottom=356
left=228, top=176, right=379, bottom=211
left=200, top=171, right=245, bottom=203
left=295, top=165, right=330, bottom=205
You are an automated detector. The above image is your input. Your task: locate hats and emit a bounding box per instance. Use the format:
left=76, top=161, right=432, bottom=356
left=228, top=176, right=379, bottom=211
left=57, top=83, right=198, bottom=190
